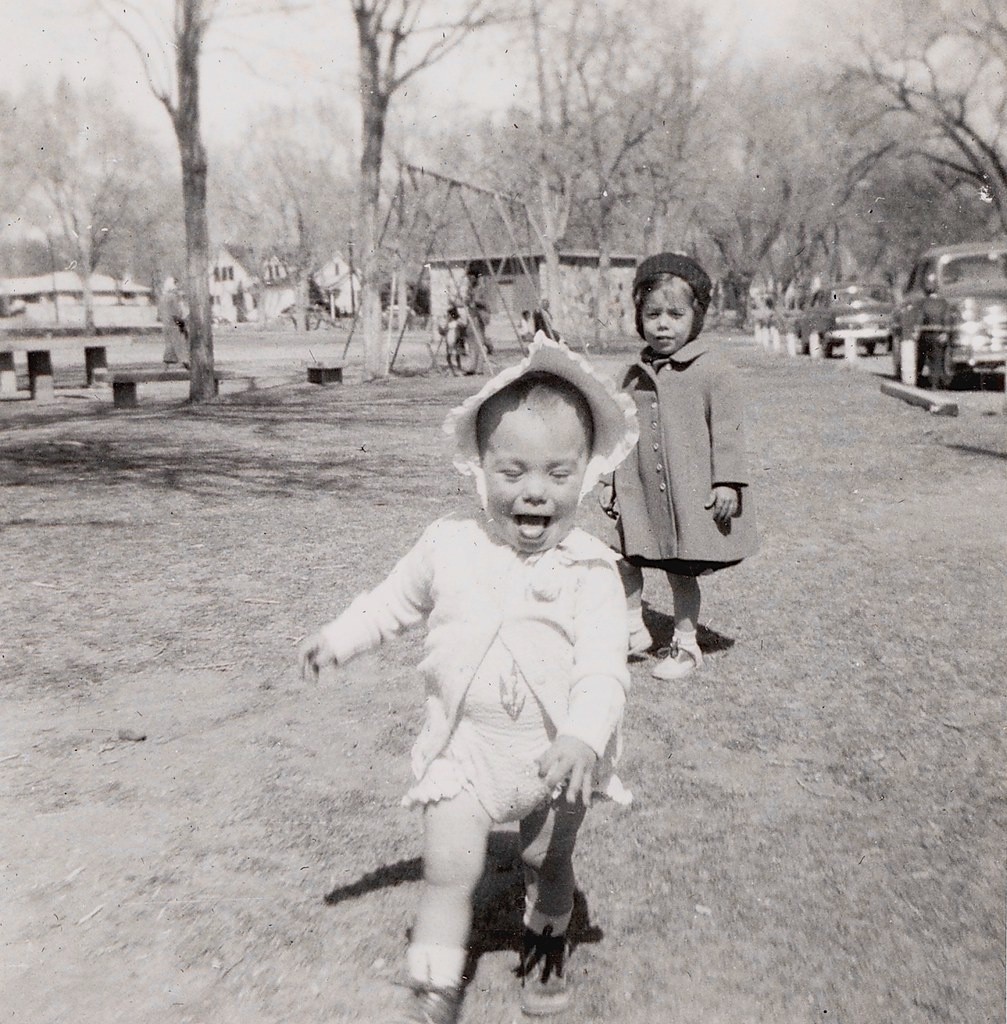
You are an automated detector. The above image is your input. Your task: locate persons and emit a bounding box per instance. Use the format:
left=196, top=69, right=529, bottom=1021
left=517, top=298, right=561, bottom=343
left=157, top=276, right=190, bottom=370
left=600, top=252, right=760, bottom=681
left=297, top=369, right=630, bottom=1024
left=435, top=267, right=493, bottom=375
left=915, top=273, right=950, bottom=390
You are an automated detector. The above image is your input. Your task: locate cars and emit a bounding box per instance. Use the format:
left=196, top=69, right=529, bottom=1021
left=799, top=283, right=898, bottom=358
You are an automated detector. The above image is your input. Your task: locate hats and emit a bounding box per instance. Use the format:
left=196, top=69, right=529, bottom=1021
left=442, top=330, right=642, bottom=524
left=634, top=252, right=712, bottom=315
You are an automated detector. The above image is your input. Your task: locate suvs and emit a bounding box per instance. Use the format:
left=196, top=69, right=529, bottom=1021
left=888, top=240, right=1007, bottom=390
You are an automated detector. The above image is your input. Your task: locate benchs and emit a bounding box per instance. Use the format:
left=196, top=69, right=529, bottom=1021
left=102, top=370, right=227, bottom=410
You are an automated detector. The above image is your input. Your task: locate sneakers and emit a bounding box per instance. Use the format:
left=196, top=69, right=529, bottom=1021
left=522, top=921, right=571, bottom=1015
left=629, top=624, right=652, bottom=655
left=652, top=643, right=702, bottom=679
left=394, top=988, right=463, bottom=1024
